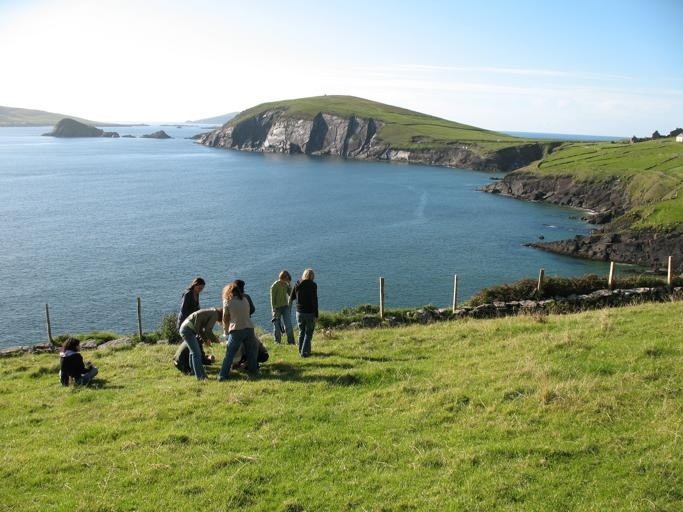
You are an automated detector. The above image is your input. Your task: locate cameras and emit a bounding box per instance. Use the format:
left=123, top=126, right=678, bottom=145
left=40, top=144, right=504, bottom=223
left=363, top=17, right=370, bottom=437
left=271, top=316, right=280, bottom=323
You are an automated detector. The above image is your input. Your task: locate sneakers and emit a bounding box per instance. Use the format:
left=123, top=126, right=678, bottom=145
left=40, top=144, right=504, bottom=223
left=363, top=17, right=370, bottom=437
left=217, top=371, right=262, bottom=381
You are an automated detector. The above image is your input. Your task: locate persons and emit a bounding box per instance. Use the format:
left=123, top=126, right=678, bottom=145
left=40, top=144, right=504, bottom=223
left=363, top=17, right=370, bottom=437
left=57, top=335, right=98, bottom=388
left=287, top=267, right=319, bottom=358
left=178, top=306, right=223, bottom=381
left=268, top=269, right=297, bottom=346
left=214, top=281, right=259, bottom=382
left=172, top=333, right=206, bottom=376
left=176, top=276, right=211, bottom=366
left=232, top=278, right=256, bottom=319
left=230, top=332, right=270, bottom=370
